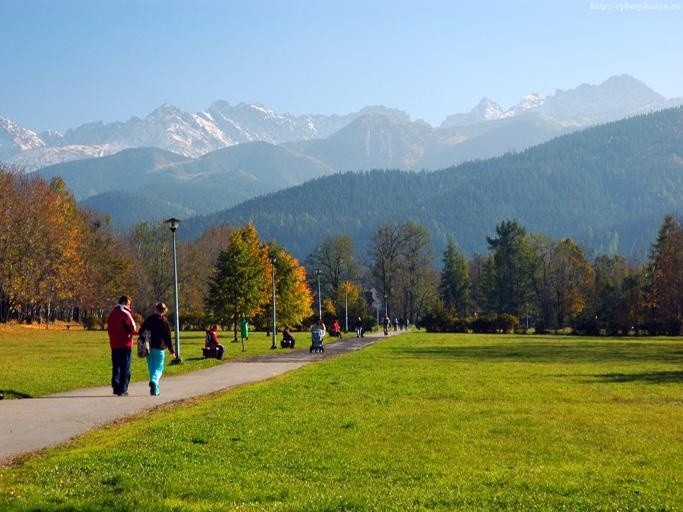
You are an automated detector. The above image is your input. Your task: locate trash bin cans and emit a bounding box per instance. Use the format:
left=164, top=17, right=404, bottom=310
left=239, top=321, right=248, bottom=340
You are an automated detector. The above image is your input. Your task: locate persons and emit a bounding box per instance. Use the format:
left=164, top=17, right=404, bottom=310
left=106, top=296, right=141, bottom=397
left=356, top=317, right=363, bottom=338
left=311, top=319, right=326, bottom=353
left=205, top=325, right=224, bottom=359
left=330, top=320, right=342, bottom=339
left=381, top=315, right=409, bottom=335
left=283, top=326, right=295, bottom=349
left=138, top=303, right=176, bottom=395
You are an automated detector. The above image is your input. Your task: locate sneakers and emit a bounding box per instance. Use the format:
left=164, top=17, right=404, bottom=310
left=113, top=389, right=128, bottom=396
left=149, top=381, right=156, bottom=395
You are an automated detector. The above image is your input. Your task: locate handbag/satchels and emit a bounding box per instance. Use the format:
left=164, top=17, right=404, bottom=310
left=137, top=330, right=152, bottom=356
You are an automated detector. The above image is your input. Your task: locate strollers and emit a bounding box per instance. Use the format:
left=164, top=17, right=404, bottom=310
left=310, top=329, right=324, bottom=354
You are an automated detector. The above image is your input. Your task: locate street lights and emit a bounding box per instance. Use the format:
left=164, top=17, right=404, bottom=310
left=267, top=251, right=277, bottom=349
left=344, top=280, right=348, bottom=333
left=315, top=268, right=321, bottom=320
left=163, top=216, right=182, bottom=365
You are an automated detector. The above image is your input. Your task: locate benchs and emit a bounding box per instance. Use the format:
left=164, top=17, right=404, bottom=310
left=280, top=339, right=295, bottom=349
left=201, top=346, right=224, bottom=360
